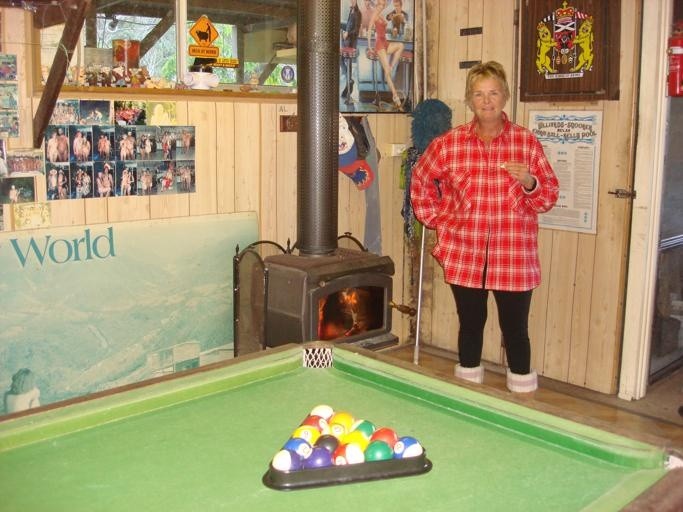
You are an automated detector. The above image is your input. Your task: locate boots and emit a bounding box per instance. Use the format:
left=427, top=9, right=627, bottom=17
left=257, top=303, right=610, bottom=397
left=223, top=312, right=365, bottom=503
left=454, top=363, right=486, bottom=388
left=503, top=368, right=539, bottom=401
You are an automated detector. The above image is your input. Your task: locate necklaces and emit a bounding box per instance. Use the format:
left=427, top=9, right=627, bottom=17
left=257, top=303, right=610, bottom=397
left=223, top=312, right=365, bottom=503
left=408, top=60, right=559, bottom=398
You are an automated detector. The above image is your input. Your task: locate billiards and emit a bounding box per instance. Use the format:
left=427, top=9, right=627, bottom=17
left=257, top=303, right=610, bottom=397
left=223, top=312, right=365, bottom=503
left=271, top=405, right=424, bottom=472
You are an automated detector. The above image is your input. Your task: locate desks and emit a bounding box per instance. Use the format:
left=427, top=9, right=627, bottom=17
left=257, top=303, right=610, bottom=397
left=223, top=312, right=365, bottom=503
left=1, top=338, right=683, bottom=511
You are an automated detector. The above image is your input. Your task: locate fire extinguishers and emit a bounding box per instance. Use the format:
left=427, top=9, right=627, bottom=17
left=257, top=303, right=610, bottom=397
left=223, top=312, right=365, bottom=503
left=666, top=21, right=683, bottom=97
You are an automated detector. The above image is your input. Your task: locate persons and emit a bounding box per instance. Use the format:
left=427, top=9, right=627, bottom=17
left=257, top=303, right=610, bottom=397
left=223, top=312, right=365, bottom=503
left=47, top=104, right=195, bottom=199
left=7, top=184, right=23, bottom=199
left=341, top=1, right=408, bottom=111
